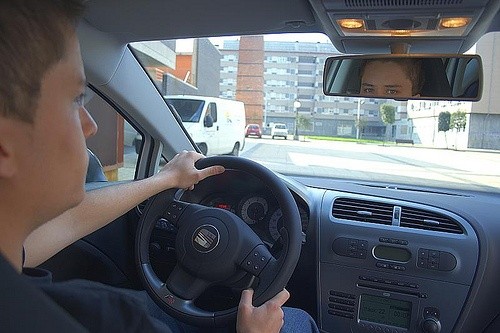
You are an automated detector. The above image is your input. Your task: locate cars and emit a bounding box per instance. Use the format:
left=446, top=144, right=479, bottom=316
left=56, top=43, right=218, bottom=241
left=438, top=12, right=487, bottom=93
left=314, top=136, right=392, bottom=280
left=271, top=122, right=288, bottom=140
left=245, top=123, right=262, bottom=138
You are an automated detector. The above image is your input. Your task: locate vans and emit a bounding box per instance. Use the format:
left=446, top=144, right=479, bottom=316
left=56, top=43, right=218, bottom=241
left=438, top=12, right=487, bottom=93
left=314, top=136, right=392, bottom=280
left=132, top=94, right=247, bottom=159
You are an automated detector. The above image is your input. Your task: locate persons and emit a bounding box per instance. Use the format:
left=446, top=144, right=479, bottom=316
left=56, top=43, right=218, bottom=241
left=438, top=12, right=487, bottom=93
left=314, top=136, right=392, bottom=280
left=0, top=0, right=319, bottom=333
left=358, top=58, right=421, bottom=99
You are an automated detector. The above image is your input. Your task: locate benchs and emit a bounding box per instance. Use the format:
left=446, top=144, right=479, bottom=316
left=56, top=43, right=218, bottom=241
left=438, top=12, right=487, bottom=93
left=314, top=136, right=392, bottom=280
left=396, top=139, right=414, bottom=145
